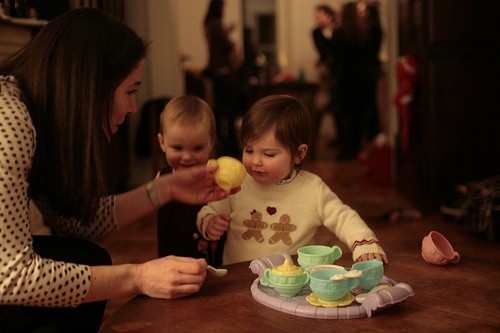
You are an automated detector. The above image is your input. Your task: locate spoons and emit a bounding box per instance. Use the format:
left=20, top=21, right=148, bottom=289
left=207, top=265, right=227, bottom=276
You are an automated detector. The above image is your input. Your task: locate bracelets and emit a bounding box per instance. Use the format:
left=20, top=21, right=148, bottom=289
left=146, top=179, right=160, bottom=209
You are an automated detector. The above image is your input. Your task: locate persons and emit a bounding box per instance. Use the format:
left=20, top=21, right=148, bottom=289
left=197, top=93, right=389, bottom=267
left=0, top=7, right=241, bottom=333
left=200, top=0, right=237, bottom=158
left=312, top=0, right=384, bottom=164
left=156, top=96, right=228, bottom=268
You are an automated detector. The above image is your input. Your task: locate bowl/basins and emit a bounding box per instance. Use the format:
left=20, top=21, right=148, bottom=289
left=264, top=258, right=310, bottom=298
left=421, top=231, right=460, bottom=267
left=309, top=267, right=358, bottom=300
left=206, top=156, right=247, bottom=190
left=352, top=259, right=384, bottom=292
left=297, top=244, right=343, bottom=271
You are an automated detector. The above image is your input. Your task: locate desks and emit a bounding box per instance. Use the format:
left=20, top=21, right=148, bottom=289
left=96, top=249, right=500, bottom=333
